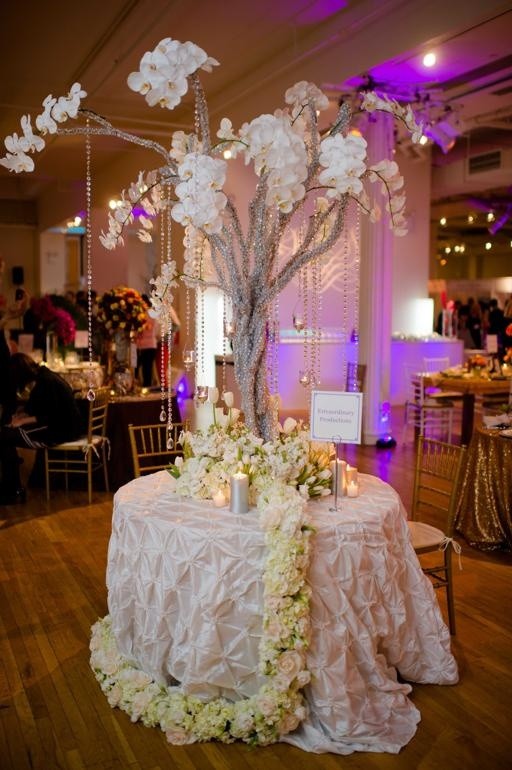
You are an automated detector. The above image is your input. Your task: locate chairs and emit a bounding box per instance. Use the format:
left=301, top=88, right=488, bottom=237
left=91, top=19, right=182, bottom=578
left=39, top=385, right=190, bottom=504
left=401, top=357, right=469, bottom=638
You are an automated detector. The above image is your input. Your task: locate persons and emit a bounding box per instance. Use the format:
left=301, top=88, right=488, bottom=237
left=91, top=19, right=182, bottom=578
left=134, top=292, right=160, bottom=386
left=435, top=296, right=511, bottom=361
left=0, top=351, right=85, bottom=505
left=157, top=300, right=181, bottom=394
left=65, top=288, right=108, bottom=365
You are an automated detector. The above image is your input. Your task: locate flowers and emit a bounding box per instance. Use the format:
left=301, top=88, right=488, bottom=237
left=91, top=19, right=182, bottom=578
left=88, top=411, right=334, bottom=748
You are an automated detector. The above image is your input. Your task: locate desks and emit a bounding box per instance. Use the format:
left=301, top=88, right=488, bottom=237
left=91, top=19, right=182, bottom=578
left=409, top=366, right=512, bottom=455
left=106, top=470, right=459, bottom=755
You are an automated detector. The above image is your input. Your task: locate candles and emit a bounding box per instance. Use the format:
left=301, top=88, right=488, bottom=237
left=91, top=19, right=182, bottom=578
left=330, top=459, right=360, bottom=498
left=229, top=472, right=248, bottom=514
